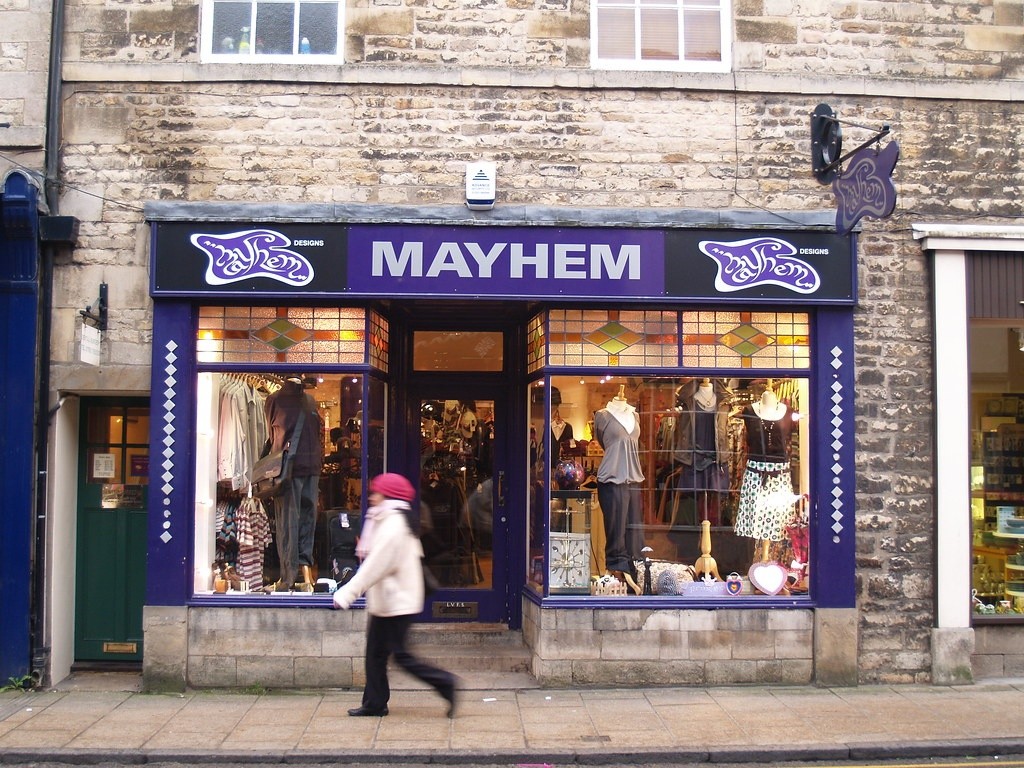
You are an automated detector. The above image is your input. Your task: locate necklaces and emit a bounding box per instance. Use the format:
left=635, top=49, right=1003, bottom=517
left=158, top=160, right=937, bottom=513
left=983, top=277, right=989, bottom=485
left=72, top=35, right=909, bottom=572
left=698, top=391, right=714, bottom=405
left=758, top=402, right=779, bottom=445
left=624, top=406, right=628, bottom=412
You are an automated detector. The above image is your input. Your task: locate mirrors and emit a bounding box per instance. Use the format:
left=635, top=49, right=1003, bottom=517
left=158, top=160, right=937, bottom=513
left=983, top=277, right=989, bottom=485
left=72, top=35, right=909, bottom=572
left=749, top=561, right=787, bottom=595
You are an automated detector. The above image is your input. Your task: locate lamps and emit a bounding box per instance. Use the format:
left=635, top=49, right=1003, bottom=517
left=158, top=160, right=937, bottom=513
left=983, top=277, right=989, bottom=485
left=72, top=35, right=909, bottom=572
left=1012, top=328, right=1024, bottom=352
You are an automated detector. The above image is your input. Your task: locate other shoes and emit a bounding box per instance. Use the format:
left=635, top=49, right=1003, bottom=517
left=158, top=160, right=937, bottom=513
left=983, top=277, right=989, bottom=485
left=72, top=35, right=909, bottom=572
left=446, top=674, right=460, bottom=718
left=348, top=707, right=389, bottom=716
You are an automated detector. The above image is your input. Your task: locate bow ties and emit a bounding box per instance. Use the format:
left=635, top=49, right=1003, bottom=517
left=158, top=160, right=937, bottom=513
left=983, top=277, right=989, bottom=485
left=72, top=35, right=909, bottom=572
left=553, top=423, right=563, bottom=429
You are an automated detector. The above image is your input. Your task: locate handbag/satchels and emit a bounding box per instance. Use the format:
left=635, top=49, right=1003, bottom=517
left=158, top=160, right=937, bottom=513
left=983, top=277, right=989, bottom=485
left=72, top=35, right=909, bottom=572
left=787, top=493, right=809, bottom=567
left=252, top=450, right=296, bottom=499
left=633, top=557, right=694, bottom=595
left=421, top=561, right=438, bottom=595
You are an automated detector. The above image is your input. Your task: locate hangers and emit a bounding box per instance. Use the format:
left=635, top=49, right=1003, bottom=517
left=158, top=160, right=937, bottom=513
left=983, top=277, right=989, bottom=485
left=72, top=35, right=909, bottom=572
left=240, top=495, right=260, bottom=513
left=222, top=372, right=284, bottom=392
left=221, top=486, right=239, bottom=503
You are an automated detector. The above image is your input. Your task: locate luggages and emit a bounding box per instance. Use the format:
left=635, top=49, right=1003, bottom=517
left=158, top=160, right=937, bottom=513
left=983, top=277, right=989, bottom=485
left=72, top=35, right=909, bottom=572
left=315, top=507, right=362, bottom=591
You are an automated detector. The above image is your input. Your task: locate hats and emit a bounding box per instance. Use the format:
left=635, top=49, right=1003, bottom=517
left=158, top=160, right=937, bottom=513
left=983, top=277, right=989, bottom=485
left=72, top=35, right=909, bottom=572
left=330, top=409, right=363, bottom=456
left=370, top=473, right=415, bottom=502
left=461, top=412, right=477, bottom=439
left=444, top=400, right=459, bottom=422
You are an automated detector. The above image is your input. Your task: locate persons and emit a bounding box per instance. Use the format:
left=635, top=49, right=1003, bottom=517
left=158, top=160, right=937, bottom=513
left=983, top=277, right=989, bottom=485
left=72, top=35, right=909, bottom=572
left=265, top=377, right=323, bottom=587
left=673, top=377, right=734, bottom=492
left=333, top=473, right=456, bottom=718
left=594, top=385, right=646, bottom=571
left=461, top=478, right=493, bottom=530
left=734, top=388, right=796, bottom=541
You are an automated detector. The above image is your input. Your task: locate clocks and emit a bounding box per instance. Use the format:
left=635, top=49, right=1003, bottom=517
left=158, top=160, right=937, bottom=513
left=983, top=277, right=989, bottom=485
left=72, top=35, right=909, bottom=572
left=549, top=530, right=592, bottom=595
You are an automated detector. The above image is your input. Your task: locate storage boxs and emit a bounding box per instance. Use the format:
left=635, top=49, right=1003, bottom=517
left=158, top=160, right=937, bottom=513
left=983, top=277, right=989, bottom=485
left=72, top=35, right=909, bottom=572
left=683, top=580, right=754, bottom=597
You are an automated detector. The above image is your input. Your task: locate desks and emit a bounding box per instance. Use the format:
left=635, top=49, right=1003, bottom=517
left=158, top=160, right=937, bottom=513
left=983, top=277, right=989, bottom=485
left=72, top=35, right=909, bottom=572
left=975, top=546, right=1018, bottom=606
